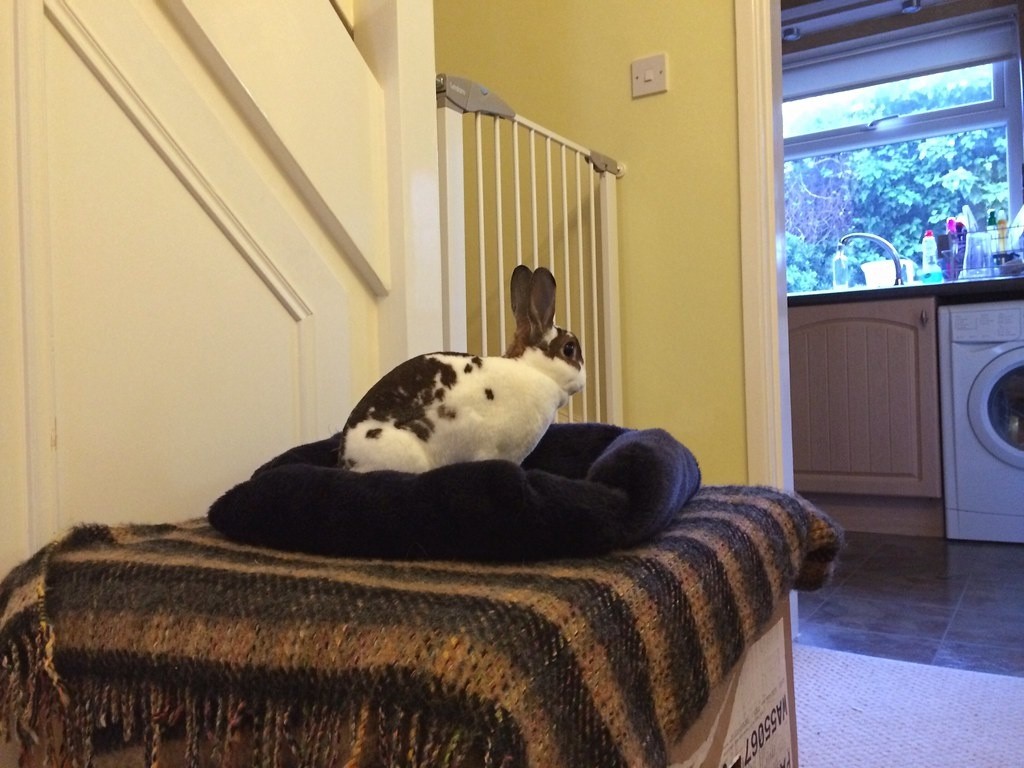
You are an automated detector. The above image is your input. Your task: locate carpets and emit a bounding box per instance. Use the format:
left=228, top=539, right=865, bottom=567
left=792, top=642, right=1024, bottom=768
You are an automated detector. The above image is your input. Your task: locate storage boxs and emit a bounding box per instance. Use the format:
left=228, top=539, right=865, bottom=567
left=33, top=487, right=798, bottom=768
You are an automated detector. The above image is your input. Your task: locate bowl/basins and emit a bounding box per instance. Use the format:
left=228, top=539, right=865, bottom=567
left=861, top=260, right=914, bottom=286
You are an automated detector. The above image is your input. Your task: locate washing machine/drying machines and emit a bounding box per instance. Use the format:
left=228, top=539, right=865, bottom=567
left=936, top=299, right=1023, bottom=543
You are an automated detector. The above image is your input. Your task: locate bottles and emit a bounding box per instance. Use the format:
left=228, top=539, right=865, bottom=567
left=946, top=219, right=958, bottom=256
left=987, top=211, right=998, bottom=252
left=997, top=210, right=1007, bottom=250
left=833, top=244, right=848, bottom=288
left=922, top=230, right=936, bottom=271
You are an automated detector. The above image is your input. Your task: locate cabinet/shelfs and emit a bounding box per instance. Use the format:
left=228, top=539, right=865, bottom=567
left=784, top=285, right=945, bottom=540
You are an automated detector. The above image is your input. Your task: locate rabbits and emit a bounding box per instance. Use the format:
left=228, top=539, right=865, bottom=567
left=336, top=265, right=587, bottom=476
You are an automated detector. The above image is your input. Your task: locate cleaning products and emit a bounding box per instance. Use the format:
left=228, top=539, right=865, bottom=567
left=918, top=210, right=1012, bottom=285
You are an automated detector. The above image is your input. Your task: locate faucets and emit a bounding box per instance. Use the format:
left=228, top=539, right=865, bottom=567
left=841, top=233, right=904, bottom=286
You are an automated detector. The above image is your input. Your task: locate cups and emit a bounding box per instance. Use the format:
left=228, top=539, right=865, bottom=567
left=963, top=234, right=993, bottom=279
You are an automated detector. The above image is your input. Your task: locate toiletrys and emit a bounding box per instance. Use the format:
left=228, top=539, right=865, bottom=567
left=833, top=243, right=848, bottom=288
